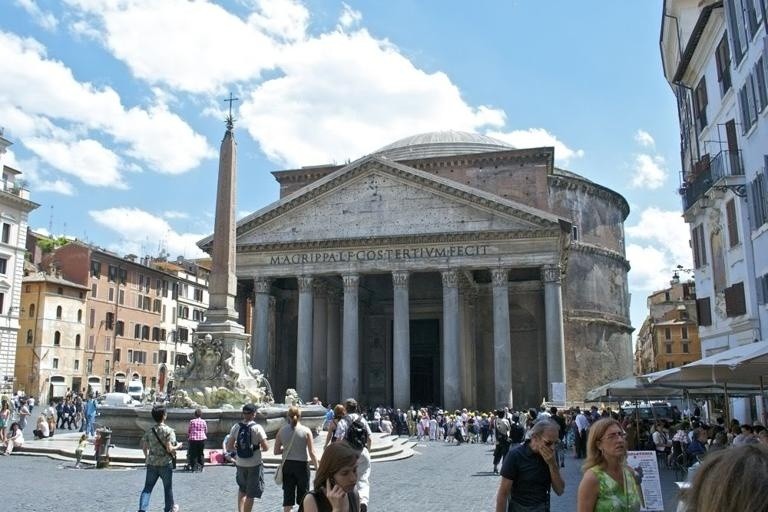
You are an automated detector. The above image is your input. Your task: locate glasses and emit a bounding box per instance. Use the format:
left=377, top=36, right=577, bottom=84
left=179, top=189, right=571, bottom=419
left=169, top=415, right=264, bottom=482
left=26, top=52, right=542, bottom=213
left=599, top=432, right=628, bottom=441
left=539, top=433, right=561, bottom=448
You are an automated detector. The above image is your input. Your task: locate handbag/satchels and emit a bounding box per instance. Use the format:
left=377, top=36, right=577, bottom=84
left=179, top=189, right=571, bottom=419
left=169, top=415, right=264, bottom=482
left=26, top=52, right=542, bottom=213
left=209, top=451, right=226, bottom=464
left=273, top=425, right=298, bottom=485
left=151, top=426, right=177, bottom=470
left=494, top=420, right=512, bottom=449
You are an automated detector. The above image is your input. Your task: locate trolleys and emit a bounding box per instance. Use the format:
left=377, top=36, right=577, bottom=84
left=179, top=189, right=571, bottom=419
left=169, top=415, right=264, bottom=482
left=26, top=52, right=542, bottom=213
left=184, top=436, right=203, bottom=473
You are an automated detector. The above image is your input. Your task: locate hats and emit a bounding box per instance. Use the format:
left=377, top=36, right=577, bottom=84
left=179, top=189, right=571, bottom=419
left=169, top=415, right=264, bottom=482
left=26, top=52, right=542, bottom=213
left=243, top=404, right=260, bottom=415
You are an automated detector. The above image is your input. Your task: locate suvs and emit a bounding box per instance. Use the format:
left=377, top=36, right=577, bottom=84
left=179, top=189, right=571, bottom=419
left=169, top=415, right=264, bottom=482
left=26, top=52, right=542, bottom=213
left=618, top=401, right=681, bottom=424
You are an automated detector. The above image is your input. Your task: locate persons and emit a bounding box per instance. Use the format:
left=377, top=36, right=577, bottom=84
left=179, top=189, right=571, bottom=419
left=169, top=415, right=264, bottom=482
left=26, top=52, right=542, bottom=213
left=300, top=439, right=359, bottom=511
left=324, top=404, right=345, bottom=450
left=325, top=404, right=335, bottom=424
left=0, top=384, right=100, bottom=468
left=495, top=415, right=566, bottom=511
left=185, top=409, right=209, bottom=473
left=333, top=398, right=372, bottom=511
left=649, top=403, right=767, bottom=469
left=576, top=417, right=640, bottom=511
left=222, top=433, right=237, bottom=465
left=356, top=403, right=649, bottom=459
left=311, top=396, right=322, bottom=405
left=680, top=444, right=766, bottom=511
left=274, top=406, right=319, bottom=511
left=234, top=402, right=268, bottom=511
left=138, top=405, right=181, bottom=511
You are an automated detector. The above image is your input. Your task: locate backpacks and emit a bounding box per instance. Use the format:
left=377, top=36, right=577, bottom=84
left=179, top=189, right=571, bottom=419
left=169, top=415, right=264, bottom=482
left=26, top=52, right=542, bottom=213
left=342, top=413, right=368, bottom=452
left=235, top=422, right=261, bottom=459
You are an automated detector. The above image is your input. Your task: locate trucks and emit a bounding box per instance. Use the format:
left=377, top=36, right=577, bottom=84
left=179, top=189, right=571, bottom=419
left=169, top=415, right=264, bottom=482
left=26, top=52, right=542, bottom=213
left=127, top=380, right=144, bottom=401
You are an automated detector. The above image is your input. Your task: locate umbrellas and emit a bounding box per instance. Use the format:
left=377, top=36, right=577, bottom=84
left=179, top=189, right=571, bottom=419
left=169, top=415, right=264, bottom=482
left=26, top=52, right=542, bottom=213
left=582, top=339, right=768, bottom=431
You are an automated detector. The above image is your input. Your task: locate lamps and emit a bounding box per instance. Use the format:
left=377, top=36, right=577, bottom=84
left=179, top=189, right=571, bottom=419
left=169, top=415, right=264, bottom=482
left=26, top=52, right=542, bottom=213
left=673, top=268, right=695, bottom=283
left=697, top=185, right=747, bottom=209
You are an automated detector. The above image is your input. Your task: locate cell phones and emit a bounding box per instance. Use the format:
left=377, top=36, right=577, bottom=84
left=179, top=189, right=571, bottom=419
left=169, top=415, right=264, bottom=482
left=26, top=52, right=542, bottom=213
left=328, top=477, right=337, bottom=490
